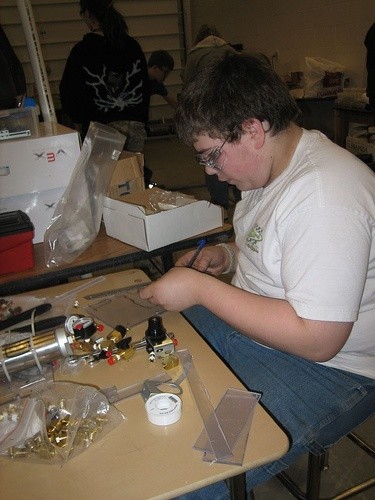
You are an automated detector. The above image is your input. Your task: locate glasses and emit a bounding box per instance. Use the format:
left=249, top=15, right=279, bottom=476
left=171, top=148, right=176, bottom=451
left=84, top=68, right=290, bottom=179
left=197, top=122, right=240, bottom=167
left=158, top=66, right=169, bottom=78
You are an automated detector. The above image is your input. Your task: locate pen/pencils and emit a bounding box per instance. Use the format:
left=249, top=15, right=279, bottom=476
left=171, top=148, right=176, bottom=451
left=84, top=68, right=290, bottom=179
left=187, top=239, right=206, bottom=268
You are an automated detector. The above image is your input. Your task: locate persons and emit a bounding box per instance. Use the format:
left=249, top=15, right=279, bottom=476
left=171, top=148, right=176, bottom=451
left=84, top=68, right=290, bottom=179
left=58, top=0, right=151, bottom=154
left=146, top=49, right=178, bottom=108
left=180, top=25, right=242, bottom=209
left=139, top=48, right=375, bottom=499
left=0, top=25, right=27, bottom=111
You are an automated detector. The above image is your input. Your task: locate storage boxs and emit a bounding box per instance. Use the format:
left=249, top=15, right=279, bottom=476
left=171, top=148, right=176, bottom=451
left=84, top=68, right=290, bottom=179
left=0, top=210, right=35, bottom=277
left=102, top=187, right=223, bottom=252
left=0, top=183, right=91, bottom=245
left=0, top=122, right=82, bottom=198
left=105, top=151, right=145, bottom=192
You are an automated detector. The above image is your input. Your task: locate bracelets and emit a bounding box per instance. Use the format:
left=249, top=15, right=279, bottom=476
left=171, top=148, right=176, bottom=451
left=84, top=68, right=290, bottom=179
left=216, top=243, right=234, bottom=275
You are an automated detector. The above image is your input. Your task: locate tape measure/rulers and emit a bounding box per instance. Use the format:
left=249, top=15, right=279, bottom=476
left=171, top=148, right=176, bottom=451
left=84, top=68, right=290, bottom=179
left=84, top=281, right=151, bottom=301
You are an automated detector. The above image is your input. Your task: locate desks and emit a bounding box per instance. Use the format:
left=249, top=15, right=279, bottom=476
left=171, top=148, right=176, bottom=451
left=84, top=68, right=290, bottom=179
left=0, top=220, right=235, bottom=293
left=332, top=104, right=375, bottom=149
left=295, top=94, right=337, bottom=142
left=0, top=269, right=289, bottom=500
